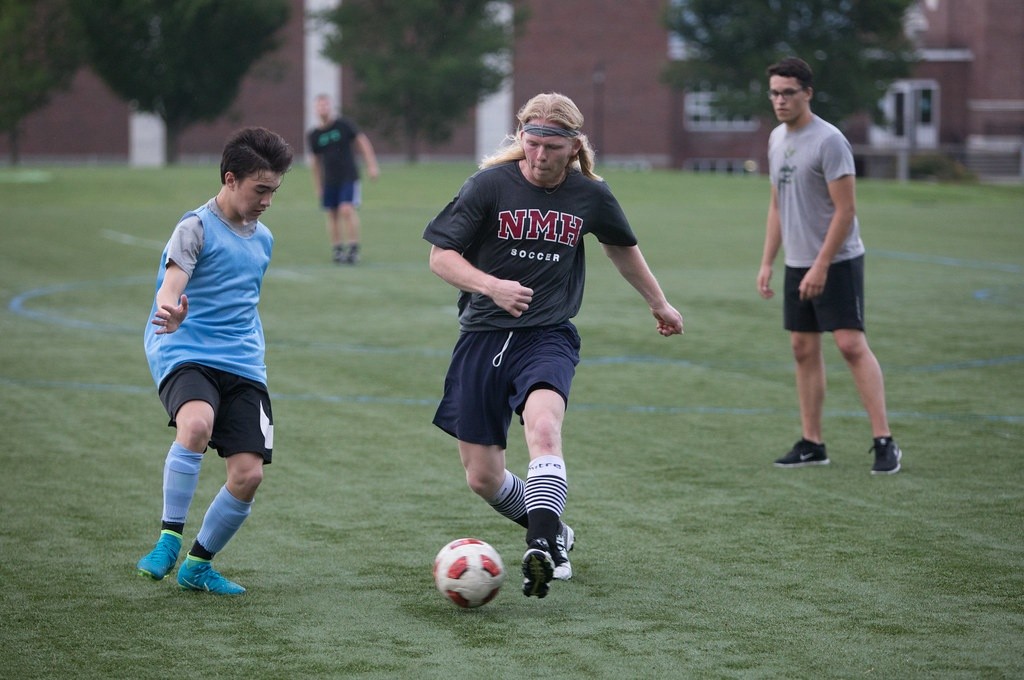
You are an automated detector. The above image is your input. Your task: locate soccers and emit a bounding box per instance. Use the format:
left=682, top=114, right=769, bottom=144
left=433, top=538, right=505, bottom=609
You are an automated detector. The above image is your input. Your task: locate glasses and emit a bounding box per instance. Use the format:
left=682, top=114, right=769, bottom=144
left=768, top=87, right=802, bottom=102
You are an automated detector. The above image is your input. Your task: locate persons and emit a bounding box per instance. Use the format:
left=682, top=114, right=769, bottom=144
left=305, top=91, right=381, bottom=266
left=422, top=93, right=685, bottom=600
left=755, top=56, right=903, bottom=476
left=137, top=129, right=295, bottom=595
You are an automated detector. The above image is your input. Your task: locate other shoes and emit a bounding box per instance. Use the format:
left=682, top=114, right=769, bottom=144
left=334, top=248, right=343, bottom=261
left=347, top=249, right=358, bottom=263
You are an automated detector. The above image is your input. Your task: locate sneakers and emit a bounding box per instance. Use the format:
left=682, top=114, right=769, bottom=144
left=773, top=436, right=830, bottom=467
left=549, top=519, right=576, bottom=581
left=177, top=557, right=247, bottom=595
left=521, top=537, right=554, bottom=599
left=136, top=533, right=182, bottom=582
left=868, top=434, right=903, bottom=476
